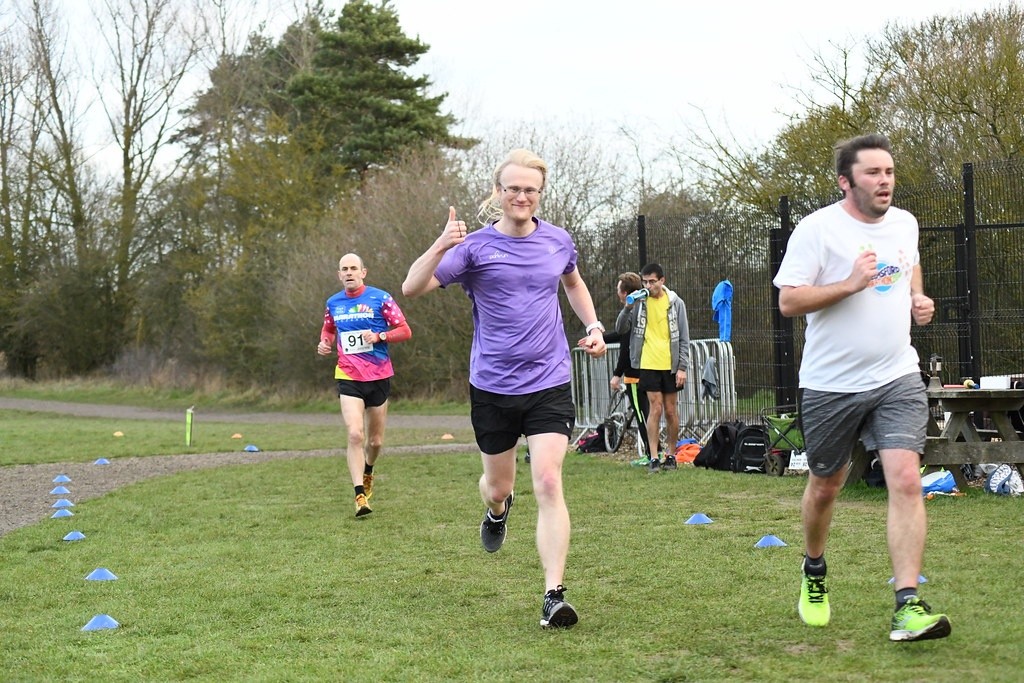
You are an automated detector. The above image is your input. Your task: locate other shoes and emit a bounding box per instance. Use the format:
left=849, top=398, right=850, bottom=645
left=525, top=451, right=530, bottom=463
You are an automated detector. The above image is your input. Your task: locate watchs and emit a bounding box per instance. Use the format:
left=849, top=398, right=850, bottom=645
left=378, top=332, right=386, bottom=344
left=586, top=321, right=606, bottom=334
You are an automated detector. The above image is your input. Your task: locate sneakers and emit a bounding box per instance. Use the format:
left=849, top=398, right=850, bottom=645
left=662, top=456, right=676, bottom=470
left=540, top=585, right=579, bottom=631
left=363, top=471, right=374, bottom=499
left=798, top=553, right=831, bottom=628
left=354, top=493, right=372, bottom=517
left=890, top=595, right=951, bottom=642
left=480, top=490, right=514, bottom=553
left=648, top=458, right=661, bottom=474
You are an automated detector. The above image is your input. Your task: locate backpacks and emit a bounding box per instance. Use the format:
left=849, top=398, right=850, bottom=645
left=730, top=424, right=771, bottom=474
left=693, top=421, right=746, bottom=470
left=575, top=418, right=616, bottom=454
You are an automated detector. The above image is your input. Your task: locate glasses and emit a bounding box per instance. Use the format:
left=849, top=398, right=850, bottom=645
left=642, top=280, right=660, bottom=286
left=498, top=182, right=542, bottom=198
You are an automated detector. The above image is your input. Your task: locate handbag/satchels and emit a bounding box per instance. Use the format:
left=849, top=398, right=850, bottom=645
left=921, top=469, right=957, bottom=498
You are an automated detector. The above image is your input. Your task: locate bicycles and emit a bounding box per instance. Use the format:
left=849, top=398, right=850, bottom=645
left=603, top=383, right=648, bottom=459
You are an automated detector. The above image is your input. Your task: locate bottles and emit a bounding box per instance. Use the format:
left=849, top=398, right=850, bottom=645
left=626, top=288, right=650, bottom=304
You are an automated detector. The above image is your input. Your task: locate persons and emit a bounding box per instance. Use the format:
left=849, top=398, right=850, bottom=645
left=615, top=261, right=689, bottom=471
left=578, top=271, right=664, bottom=466
left=318, top=252, right=413, bottom=518
left=770, top=133, right=953, bottom=642
left=402, top=149, right=608, bottom=627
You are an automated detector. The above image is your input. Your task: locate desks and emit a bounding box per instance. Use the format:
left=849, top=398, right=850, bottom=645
left=920, top=388, right=1024, bottom=494
